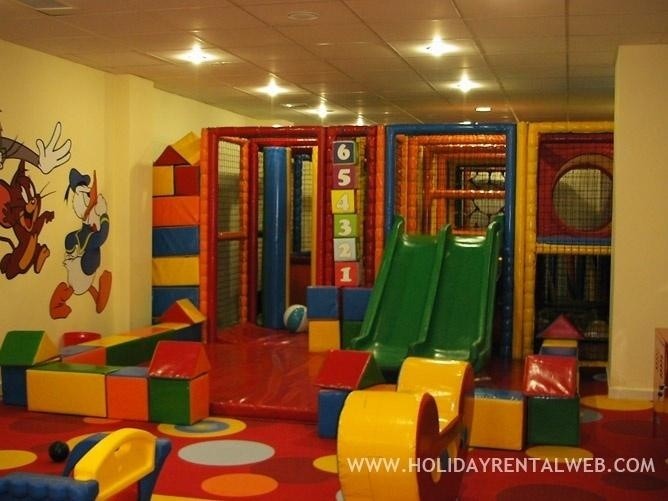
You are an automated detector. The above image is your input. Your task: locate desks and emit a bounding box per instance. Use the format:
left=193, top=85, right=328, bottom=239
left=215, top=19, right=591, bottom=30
left=653, top=327, right=667, bottom=438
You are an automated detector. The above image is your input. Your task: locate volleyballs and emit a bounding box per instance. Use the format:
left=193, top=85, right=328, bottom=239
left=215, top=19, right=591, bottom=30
left=283, top=304, right=308, bottom=334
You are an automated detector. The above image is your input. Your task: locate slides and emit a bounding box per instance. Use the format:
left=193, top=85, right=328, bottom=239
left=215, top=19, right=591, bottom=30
left=350, top=216, right=506, bottom=372
